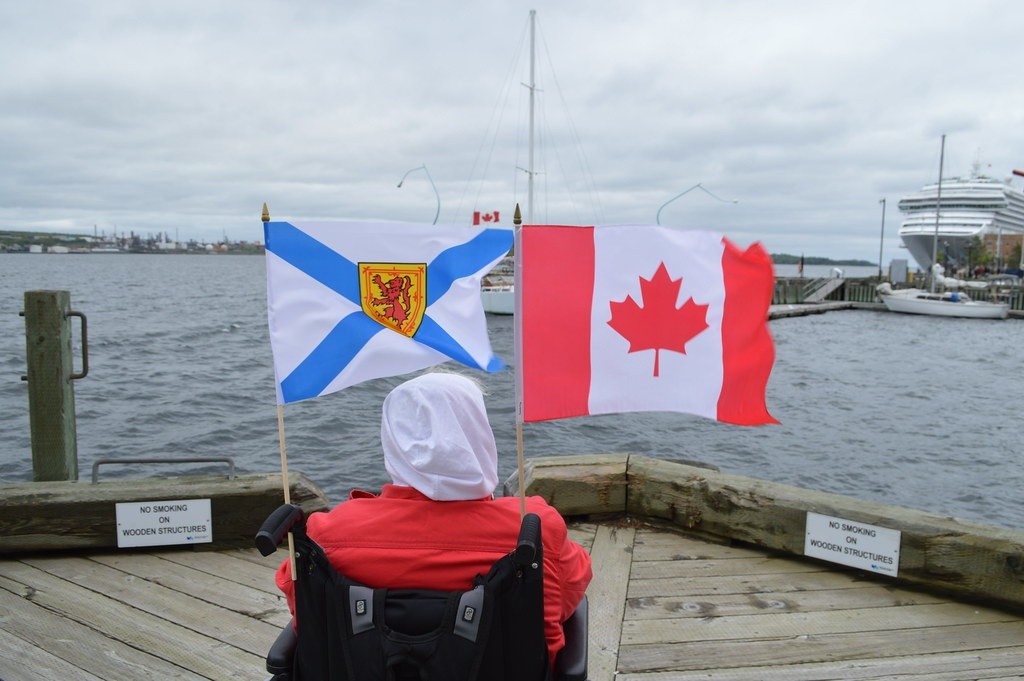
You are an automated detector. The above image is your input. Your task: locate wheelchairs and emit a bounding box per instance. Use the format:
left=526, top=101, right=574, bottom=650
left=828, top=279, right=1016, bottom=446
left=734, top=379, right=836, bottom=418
left=255, top=502, right=590, bottom=681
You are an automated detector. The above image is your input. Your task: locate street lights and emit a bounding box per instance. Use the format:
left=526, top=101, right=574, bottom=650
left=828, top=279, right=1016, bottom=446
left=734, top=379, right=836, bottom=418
left=655, top=183, right=740, bottom=225
left=397, top=162, right=441, bottom=225
left=878, top=198, right=886, bottom=282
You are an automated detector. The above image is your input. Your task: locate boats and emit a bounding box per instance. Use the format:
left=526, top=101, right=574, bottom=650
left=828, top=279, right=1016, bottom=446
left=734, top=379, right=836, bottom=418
left=897, top=173, right=1024, bottom=273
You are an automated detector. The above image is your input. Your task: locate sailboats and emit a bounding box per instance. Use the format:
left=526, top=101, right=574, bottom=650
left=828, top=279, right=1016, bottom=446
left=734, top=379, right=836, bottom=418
left=876, top=132, right=1013, bottom=320
left=479, top=7, right=605, bottom=317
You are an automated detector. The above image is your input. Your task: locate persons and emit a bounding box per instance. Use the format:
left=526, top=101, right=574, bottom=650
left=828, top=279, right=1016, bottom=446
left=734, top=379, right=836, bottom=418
left=275, top=373, right=593, bottom=672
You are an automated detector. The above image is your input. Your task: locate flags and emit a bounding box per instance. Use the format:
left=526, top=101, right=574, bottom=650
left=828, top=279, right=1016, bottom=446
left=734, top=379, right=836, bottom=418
left=512, top=225, right=783, bottom=427
left=263, top=221, right=515, bottom=405
left=474, top=212, right=498, bottom=227
